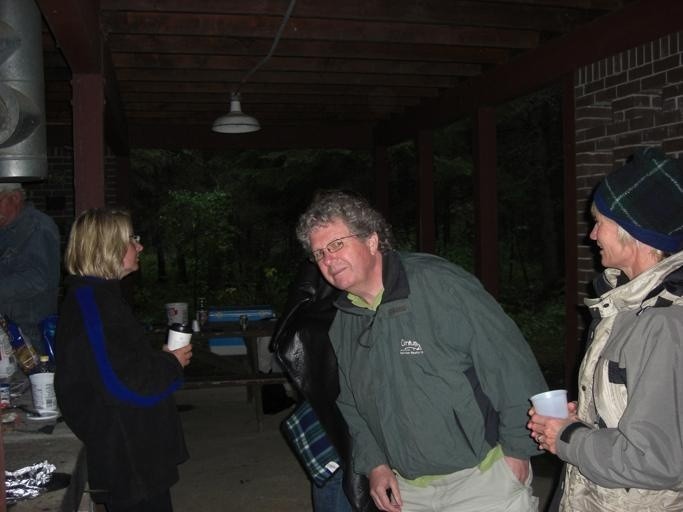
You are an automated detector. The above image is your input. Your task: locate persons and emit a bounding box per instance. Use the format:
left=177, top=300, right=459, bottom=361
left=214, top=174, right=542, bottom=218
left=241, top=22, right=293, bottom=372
left=296, top=187, right=550, bottom=512
left=54, top=205, right=192, bottom=512
left=526, top=149, right=683, bottom=512
left=0, top=183, right=61, bottom=356
left=269, top=261, right=391, bottom=512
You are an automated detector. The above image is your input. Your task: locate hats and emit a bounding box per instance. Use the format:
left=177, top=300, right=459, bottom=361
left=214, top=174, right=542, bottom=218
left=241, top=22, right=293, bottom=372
left=592, top=148, right=680, bottom=257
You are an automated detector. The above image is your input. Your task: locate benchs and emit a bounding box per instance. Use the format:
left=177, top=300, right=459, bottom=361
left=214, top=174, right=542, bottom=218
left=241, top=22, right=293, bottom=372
left=173, top=372, right=293, bottom=423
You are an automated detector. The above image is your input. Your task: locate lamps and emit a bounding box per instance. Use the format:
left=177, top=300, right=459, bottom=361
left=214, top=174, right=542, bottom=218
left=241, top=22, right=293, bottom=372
left=209, top=91, right=263, bottom=135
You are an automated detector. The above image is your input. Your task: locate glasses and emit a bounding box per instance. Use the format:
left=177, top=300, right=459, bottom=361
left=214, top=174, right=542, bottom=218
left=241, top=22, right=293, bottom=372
left=307, top=232, right=369, bottom=263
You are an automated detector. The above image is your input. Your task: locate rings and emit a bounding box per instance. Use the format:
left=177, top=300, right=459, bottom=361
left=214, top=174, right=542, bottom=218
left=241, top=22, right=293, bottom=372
left=535, top=433, right=543, bottom=443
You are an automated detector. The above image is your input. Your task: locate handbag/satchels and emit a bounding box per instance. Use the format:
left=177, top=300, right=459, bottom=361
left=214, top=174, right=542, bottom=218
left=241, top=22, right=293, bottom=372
left=279, top=398, right=346, bottom=488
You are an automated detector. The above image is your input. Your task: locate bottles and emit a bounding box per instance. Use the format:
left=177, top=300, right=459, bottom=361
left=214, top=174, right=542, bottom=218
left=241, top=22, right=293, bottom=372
left=27, top=352, right=50, bottom=373
left=0, top=384, right=10, bottom=405
left=239, top=314, right=250, bottom=333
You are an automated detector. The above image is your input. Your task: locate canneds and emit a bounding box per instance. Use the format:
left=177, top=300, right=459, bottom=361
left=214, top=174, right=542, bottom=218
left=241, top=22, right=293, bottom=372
left=240, top=315, right=249, bottom=331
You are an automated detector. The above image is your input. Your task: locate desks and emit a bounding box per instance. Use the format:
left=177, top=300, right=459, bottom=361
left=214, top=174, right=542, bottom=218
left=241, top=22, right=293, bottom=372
left=141, top=318, right=297, bottom=421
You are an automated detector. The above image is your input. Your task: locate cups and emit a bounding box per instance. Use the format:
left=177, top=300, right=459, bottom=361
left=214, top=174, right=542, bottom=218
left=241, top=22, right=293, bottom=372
left=529, top=389, right=570, bottom=421
left=164, top=301, right=190, bottom=324
left=29, top=371, right=60, bottom=413
left=166, top=320, right=194, bottom=354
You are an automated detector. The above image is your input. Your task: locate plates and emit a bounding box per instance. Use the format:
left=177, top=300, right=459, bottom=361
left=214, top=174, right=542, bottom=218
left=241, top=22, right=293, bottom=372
left=26, top=411, right=60, bottom=420
